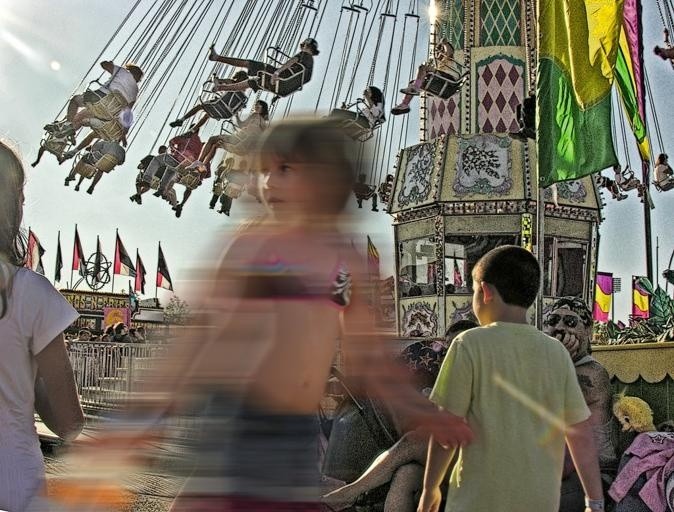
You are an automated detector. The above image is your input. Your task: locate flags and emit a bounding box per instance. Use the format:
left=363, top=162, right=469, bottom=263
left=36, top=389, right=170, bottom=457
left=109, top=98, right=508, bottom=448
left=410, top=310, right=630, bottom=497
left=367, top=233, right=383, bottom=278
left=21, top=223, right=177, bottom=295
left=592, top=272, right=614, bottom=323
left=632, top=277, right=650, bottom=321
left=350, top=236, right=363, bottom=265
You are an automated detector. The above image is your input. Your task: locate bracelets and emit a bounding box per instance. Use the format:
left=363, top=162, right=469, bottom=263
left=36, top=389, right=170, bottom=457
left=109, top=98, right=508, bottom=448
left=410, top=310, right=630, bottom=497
left=583, top=495, right=605, bottom=512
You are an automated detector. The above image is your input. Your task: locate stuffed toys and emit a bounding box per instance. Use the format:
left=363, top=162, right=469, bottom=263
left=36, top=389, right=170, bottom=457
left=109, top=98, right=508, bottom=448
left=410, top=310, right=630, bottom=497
left=613, top=387, right=657, bottom=433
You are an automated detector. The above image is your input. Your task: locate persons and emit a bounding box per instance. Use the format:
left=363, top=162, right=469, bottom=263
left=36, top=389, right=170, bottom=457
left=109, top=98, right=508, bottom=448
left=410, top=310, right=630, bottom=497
left=654, top=153, right=674, bottom=192
left=31, top=119, right=76, bottom=167
left=416, top=244, right=607, bottom=512
left=66, top=61, right=143, bottom=136
left=613, top=161, right=644, bottom=204
left=0, top=139, right=88, bottom=512
left=130, top=70, right=270, bottom=218
left=653, top=27, right=673, bottom=63
left=209, top=38, right=320, bottom=97
left=377, top=175, right=394, bottom=203
left=352, top=175, right=378, bottom=211
left=361, top=85, right=385, bottom=123
left=71, top=112, right=477, bottom=510
left=65, top=139, right=125, bottom=196
left=322, top=294, right=612, bottom=512
left=598, top=172, right=629, bottom=201
left=391, top=39, right=456, bottom=115
